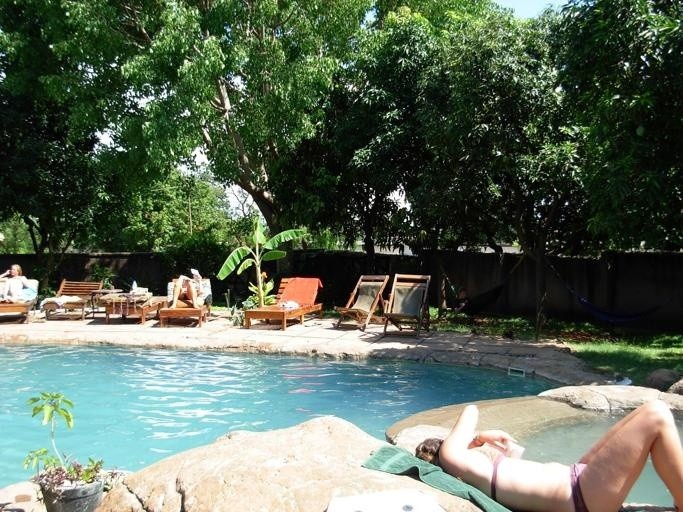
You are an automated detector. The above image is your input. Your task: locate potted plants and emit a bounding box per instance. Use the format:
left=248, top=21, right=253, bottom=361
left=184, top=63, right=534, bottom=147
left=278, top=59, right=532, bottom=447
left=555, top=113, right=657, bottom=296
left=22, top=390, right=118, bottom=511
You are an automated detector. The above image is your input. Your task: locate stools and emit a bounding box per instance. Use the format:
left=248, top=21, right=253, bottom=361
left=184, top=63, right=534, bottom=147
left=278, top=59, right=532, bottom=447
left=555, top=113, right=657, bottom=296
left=88, top=287, right=124, bottom=324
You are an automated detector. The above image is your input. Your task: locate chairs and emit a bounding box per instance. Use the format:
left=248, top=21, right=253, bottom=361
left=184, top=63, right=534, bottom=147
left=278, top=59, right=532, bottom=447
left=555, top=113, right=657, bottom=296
left=383, top=267, right=434, bottom=340
left=105, top=294, right=163, bottom=325
left=240, top=276, right=327, bottom=330
left=41, top=270, right=105, bottom=328
left=1, top=274, right=41, bottom=325
left=333, top=272, right=390, bottom=333
left=159, top=277, right=219, bottom=326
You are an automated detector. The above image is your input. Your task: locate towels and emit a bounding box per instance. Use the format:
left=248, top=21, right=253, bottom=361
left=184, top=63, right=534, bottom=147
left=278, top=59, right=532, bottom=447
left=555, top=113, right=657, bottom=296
left=359, top=446, right=512, bottom=512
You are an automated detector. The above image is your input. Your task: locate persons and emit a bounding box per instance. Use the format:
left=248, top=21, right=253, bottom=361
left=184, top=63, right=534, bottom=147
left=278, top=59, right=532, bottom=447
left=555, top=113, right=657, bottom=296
left=0, top=263, right=37, bottom=304
left=166, top=273, right=207, bottom=310
left=413, top=398, right=683, bottom=512
